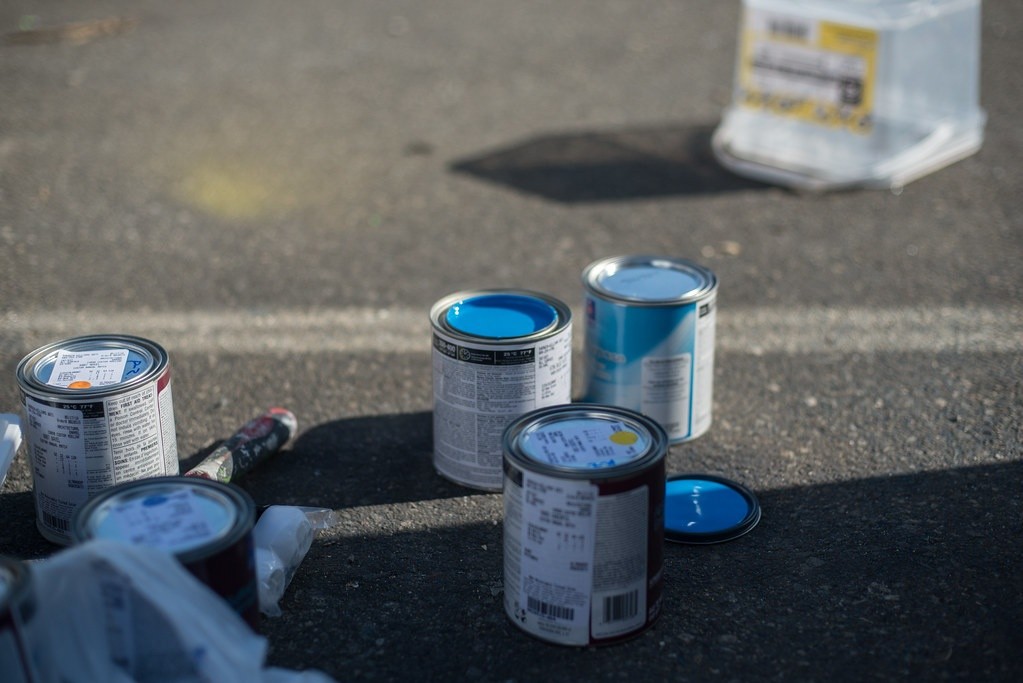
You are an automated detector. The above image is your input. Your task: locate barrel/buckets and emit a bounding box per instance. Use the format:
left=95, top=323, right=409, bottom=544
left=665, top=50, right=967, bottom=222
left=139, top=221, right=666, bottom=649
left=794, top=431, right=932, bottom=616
left=74, top=476, right=259, bottom=653
left=500, top=400, right=667, bottom=648
left=17, top=332, right=181, bottom=548
left=428, top=284, right=575, bottom=496
left=583, top=252, right=720, bottom=443
